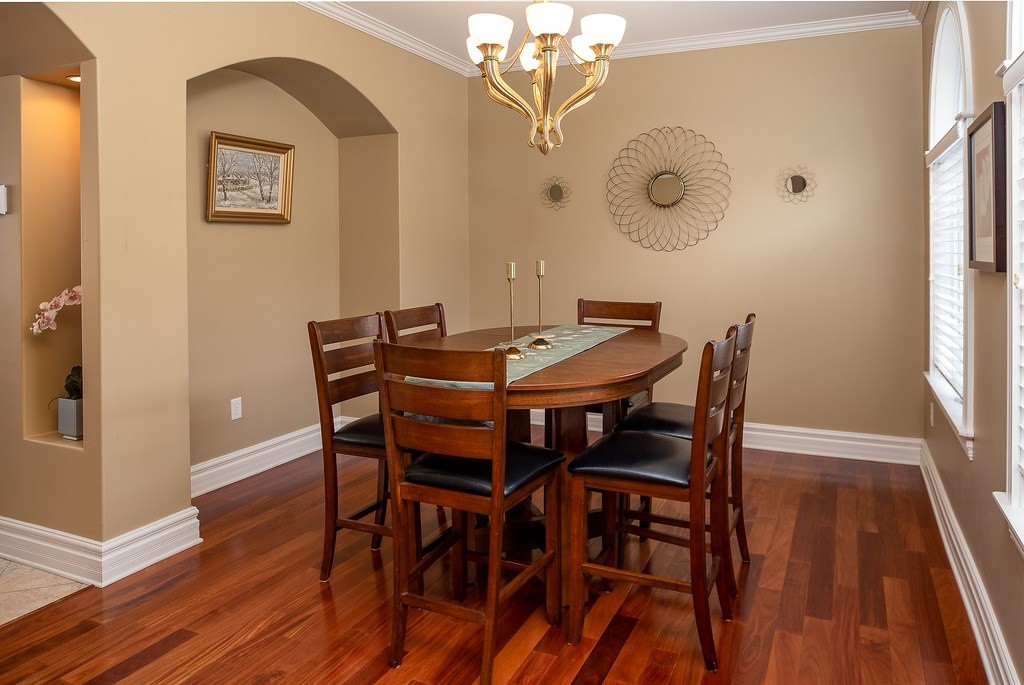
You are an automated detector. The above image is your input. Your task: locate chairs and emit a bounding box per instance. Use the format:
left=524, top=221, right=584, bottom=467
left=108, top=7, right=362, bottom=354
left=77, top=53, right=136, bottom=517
left=611, top=312, right=757, bottom=568
left=309, top=311, right=424, bottom=611
left=383, top=303, right=494, bottom=523
left=577, top=297, right=662, bottom=419
left=372, top=339, right=562, bottom=685
left=566, top=325, right=739, bottom=670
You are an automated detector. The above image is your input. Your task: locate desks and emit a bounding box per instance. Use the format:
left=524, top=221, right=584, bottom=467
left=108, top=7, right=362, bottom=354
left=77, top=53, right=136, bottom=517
left=404, top=325, right=691, bottom=629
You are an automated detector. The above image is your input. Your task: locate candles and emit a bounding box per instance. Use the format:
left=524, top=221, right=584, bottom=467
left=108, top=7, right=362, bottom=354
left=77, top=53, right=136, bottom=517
left=505, top=261, right=515, bottom=278
left=535, top=259, right=544, bottom=276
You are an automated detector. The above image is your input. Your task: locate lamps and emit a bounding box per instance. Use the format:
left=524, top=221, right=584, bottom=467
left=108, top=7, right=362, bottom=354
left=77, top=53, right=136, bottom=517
left=465, top=0, right=626, bottom=155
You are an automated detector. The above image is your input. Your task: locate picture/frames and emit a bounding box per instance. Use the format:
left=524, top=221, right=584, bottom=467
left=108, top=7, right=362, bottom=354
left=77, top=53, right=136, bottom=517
left=968, top=100, right=1004, bottom=274
left=206, top=129, right=293, bottom=226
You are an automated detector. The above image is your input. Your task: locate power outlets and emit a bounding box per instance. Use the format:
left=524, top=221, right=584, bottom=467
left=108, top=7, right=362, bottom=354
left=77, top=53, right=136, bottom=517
left=231, top=397, right=242, bottom=421
